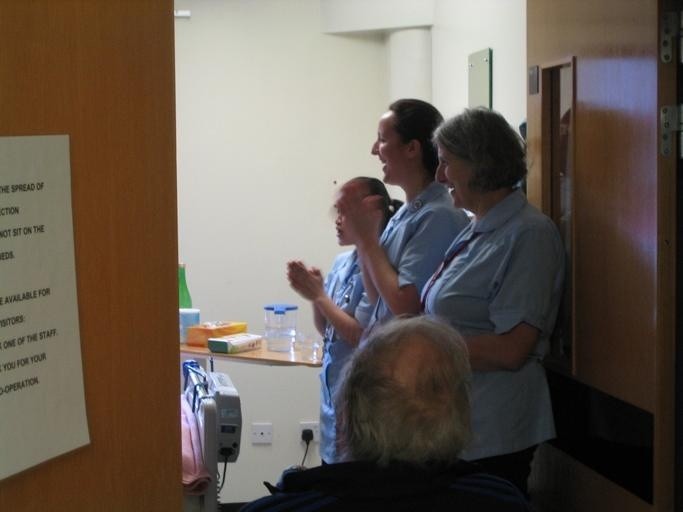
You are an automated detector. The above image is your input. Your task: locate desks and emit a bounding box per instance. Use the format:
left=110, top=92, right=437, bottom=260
left=181, top=336, right=326, bottom=371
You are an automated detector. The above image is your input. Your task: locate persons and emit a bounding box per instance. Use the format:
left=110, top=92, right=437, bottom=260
left=333, top=100, right=468, bottom=348
left=230, top=317, right=528, bottom=512
left=283, top=176, right=404, bottom=466
left=419, top=109, right=566, bottom=501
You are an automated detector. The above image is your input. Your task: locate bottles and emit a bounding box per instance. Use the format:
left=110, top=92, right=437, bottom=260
left=178, top=263, right=192, bottom=308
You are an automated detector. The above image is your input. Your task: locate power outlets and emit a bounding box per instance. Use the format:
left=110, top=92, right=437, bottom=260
left=299, top=421, right=320, bottom=443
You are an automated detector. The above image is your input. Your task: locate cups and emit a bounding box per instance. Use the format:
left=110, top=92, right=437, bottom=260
left=298, top=338, right=319, bottom=362
left=265, top=304, right=296, bottom=351
